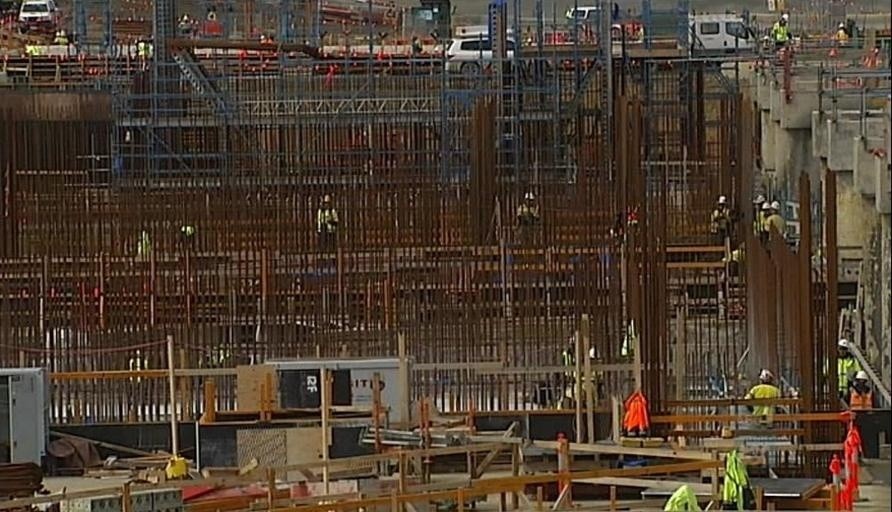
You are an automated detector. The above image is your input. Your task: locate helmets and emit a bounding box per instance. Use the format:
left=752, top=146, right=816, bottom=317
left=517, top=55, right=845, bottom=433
left=838, top=338, right=849, bottom=348
left=524, top=191, right=535, bottom=200
left=716, top=192, right=781, bottom=211
left=855, top=370, right=869, bottom=381
left=758, top=368, right=774, bottom=383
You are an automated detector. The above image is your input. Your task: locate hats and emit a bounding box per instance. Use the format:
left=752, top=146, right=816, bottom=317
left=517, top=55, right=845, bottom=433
left=782, top=13, right=789, bottom=22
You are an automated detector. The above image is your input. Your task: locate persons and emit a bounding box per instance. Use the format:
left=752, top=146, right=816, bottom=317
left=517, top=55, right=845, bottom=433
left=772, top=14, right=792, bottom=50
left=146, top=39, right=154, bottom=68
left=837, top=22, right=848, bottom=44
left=745, top=369, right=782, bottom=428
left=59, top=30, right=70, bottom=61
left=138, top=40, right=146, bottom=68
left=181, top=224, right=194, bottom=247
left=54, top=32, right=60, bottom=44
left=710, top=196, right=791, bottom=279
left=843, top=372, right=878, bottom=411
left=824, top=340, right=860, bottom=406
left=618, top=212, right=638, bottom=238
left=317, top=196, right=340, bottom=254
left=517, top=192, right=541, bottom=227
left=129, top=350, right=149, bottom=385
left=137, top=227, right=151, bottom=257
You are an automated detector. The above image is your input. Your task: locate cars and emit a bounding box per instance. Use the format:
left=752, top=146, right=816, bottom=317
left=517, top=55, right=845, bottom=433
left=567, top=10, right=644, bottom=41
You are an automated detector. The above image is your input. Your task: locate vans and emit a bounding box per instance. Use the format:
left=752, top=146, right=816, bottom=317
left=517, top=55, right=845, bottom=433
left=17, top=1, right=60, bottom=35
left=687, top=13, right=760, bottom=54
left=566, top=6, right=600, bottom=19
left=442, top=38, right=516, bottom=80
